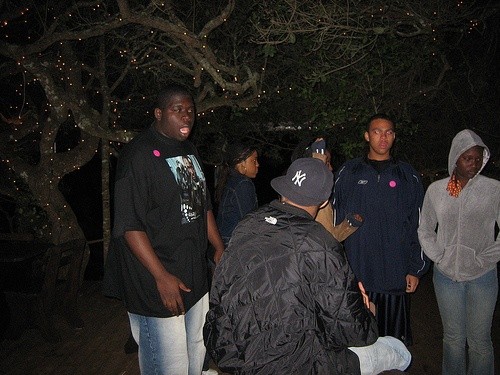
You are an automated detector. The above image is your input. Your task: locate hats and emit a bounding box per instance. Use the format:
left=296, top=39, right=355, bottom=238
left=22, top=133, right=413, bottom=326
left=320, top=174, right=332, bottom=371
left=270, top=158, right=334, bottom=206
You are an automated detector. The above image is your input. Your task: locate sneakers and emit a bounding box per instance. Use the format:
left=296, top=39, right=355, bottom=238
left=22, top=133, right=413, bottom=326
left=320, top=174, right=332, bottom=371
left=124, top=332, right=138, bottom=354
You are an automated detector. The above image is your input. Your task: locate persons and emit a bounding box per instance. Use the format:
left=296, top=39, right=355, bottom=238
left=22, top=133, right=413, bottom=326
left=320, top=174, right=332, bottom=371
left=206, top=146, right=259, bottom=288
left=202, top=158, right=412, bottom=375
left=333, top=113, right=434, bottom=346
left=103, top=85, right=223, bottom=375
left=291, top=137, right=363, bottom=243
left=418, top=129, right=500, bottom=375
